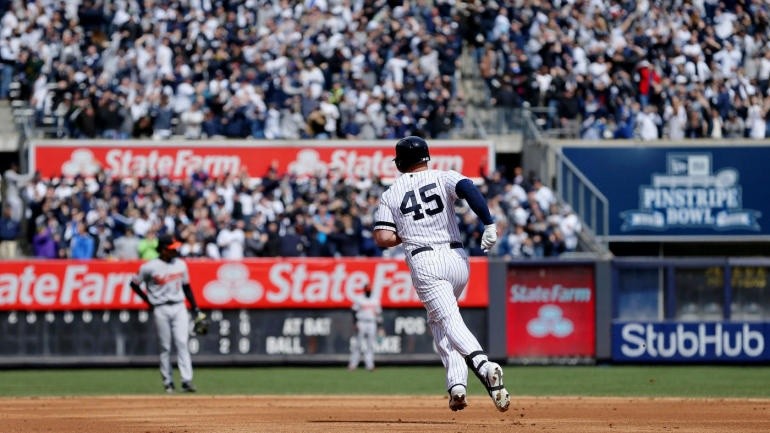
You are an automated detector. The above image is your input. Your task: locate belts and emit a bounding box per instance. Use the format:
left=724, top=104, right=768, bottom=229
left=412, top=242, right=462, bottom=256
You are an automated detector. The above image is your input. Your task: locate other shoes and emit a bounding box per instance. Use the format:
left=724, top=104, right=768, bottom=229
left=165, top=382, right=175, bottom=393
left=181, top=383, right=197, bottom=393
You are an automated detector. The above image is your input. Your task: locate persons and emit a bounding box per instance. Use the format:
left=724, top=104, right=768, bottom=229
left=0, top=0, right=770, bottom=261
left=350, top=284, right=384, bottom=371
left=374, top=136, right=512, bottom=414
left=131, top=235, right=209, bottom=393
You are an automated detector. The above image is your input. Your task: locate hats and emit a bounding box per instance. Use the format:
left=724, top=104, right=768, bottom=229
left=158, top=235, right=182, bottom=250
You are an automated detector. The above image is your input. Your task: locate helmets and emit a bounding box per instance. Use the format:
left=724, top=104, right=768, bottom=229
left=392, top=136, right=430, bottom=171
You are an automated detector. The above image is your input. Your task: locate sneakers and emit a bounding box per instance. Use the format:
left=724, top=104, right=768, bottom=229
left=485, top=363, right=510, bottom=412
left=449, top=385, right=467, bottom=411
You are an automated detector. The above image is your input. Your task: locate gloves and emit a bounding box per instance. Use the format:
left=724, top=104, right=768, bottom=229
left=481, top=223, right=497, bottom=250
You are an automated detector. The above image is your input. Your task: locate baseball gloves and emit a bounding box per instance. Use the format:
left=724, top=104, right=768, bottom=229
left=195, top=312, right=208, bottom=336
